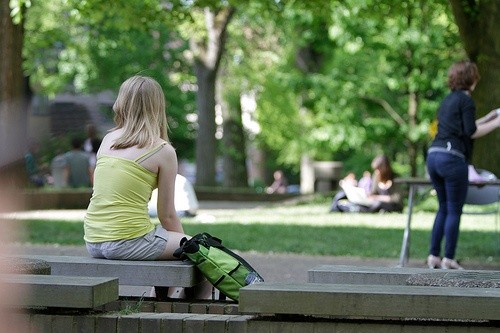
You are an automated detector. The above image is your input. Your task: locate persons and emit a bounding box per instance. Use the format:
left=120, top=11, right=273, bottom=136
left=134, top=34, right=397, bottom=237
left=426, top=60, right=500, bottom=269
left=23, top=122, right=102, bottom=190
left=329, top=153, right=405, bottom=214
left=83, top=75, right=214, bottom=299
left=265, top=169, right=289, bottom=194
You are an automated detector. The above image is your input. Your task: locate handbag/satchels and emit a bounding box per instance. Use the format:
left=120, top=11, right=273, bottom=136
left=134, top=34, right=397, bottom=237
left=173, top=232, right=264, bottom=304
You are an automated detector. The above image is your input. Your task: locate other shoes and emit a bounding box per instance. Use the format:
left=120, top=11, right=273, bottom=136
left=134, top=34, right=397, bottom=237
left=427, top=254, right=441, bottom=269
left=441, top=257, right=463, bottom=269
